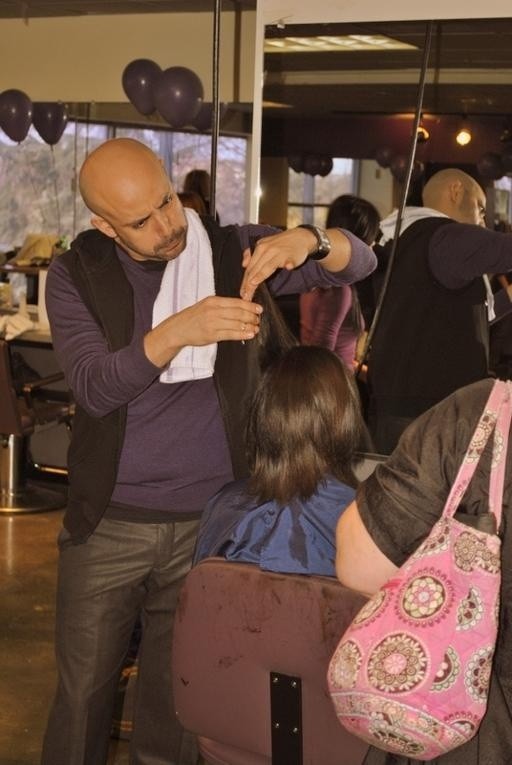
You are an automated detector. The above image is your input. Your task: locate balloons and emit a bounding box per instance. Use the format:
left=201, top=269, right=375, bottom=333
left=120, top=59, right=164, bottom=117
left=1, top=89, right=34, bottom=144
left=155, top=66, right=204, bottom=127
left=33, top=101, right=69, bottom=147
left=193, top=102, right=228, bottom=135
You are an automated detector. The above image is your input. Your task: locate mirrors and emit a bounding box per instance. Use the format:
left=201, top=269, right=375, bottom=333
left=1, top=102, right=251, bottom=349
left=257, top=16, right=511, bottom=461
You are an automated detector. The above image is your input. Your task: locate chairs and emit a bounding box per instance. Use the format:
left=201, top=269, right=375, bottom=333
left=173, top=557, right=371, bottom=765
left=0, top=338, right=67, bottom=514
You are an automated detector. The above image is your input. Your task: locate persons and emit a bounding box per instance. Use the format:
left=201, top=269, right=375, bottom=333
left=183, top=169, right=221, bottom=226
left=360, top=165, right=511, bottom=454
left=175, top=191, right=204, bottom=219
left=302, top=195, right=378, bottom=375
left=178, top=343, right=378, bottom=598
left=44, top=134, right=379, bottom=761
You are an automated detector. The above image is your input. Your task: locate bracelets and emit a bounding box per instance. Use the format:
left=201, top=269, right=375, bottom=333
left=298, top=223, right=331, bottom=263
